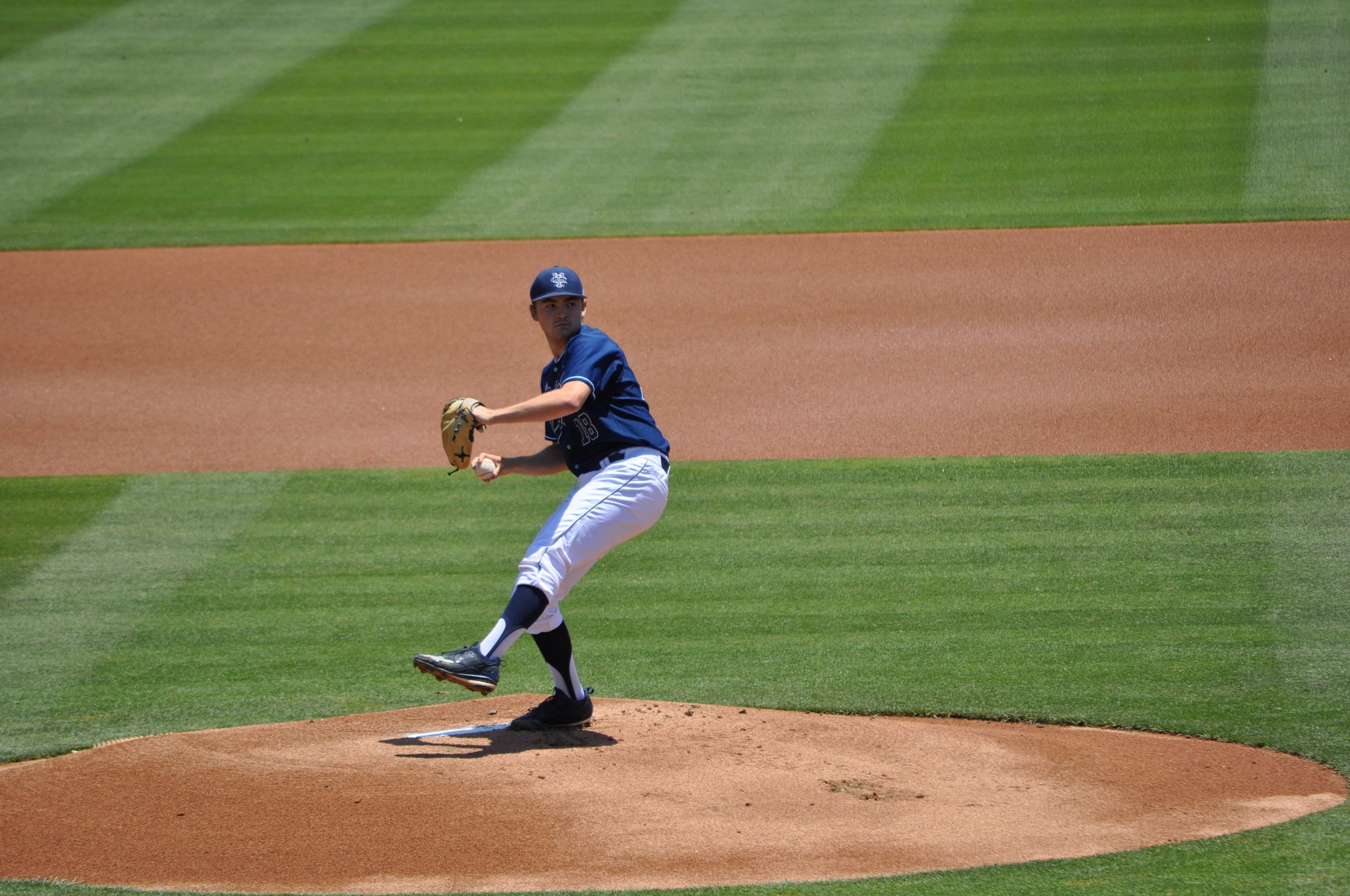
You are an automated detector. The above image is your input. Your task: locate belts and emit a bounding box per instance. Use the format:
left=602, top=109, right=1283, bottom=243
left=576, top=453, right=671, bottom=473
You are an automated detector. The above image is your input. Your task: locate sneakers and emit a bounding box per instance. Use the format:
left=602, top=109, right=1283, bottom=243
left=511, top=686, right=595, bottom=731
left=413, top=644, right=507, bottom=696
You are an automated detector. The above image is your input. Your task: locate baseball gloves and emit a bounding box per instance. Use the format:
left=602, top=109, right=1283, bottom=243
left=440, top=397, right=485, bottom=472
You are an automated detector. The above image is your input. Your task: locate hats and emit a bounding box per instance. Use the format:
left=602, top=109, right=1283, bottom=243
left=529, top=266, right=588, bottom=302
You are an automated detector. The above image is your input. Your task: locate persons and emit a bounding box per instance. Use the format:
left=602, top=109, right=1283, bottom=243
left=415, top=265, right=671, bottom=732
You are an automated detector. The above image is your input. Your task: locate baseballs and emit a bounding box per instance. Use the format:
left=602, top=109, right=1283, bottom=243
left=475, top=458, right=495, bottom=480
